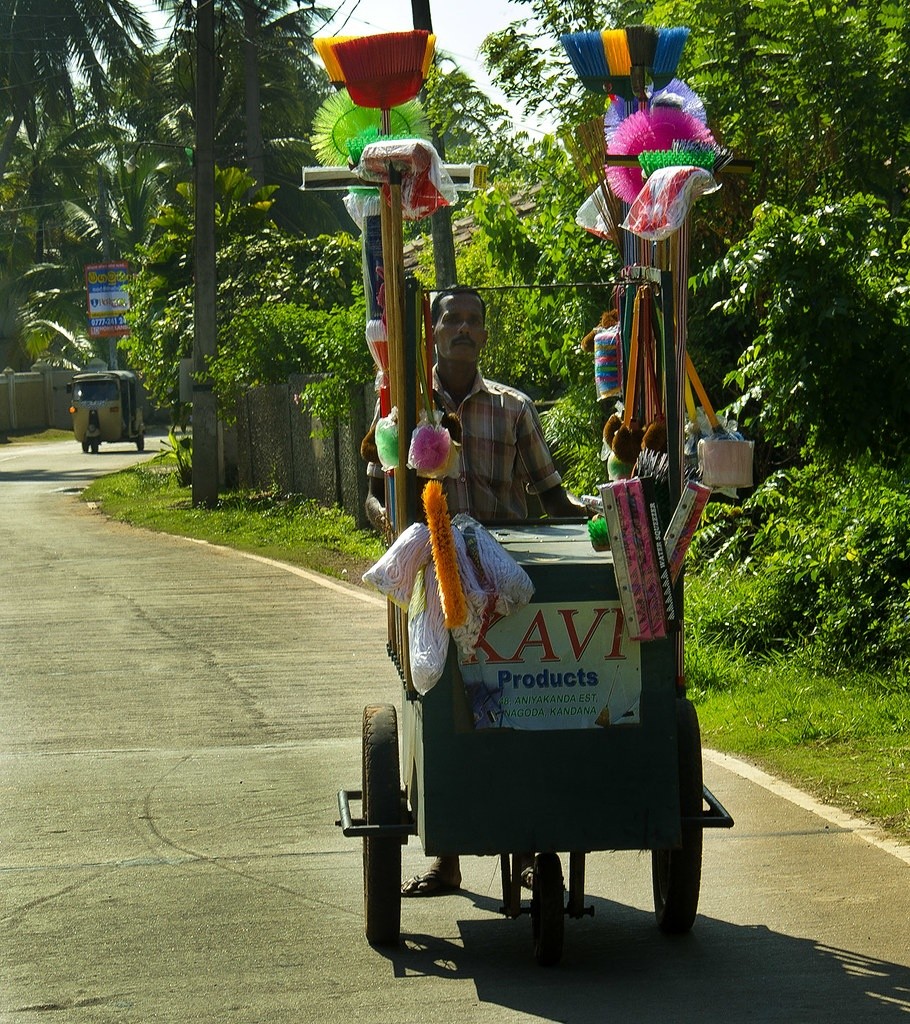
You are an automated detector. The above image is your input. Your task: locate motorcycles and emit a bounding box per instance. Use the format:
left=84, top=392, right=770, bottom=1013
left=68, top=371, right=149, bottom=455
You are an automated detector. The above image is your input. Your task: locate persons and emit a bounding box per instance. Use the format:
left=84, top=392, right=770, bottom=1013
left=360, top=282, right=597, bottom=897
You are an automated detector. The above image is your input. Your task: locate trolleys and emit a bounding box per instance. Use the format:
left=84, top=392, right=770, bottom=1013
left=334, top=526, right=735, bottom=964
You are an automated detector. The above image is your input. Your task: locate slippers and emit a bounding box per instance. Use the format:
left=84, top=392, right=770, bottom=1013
left=401, top=875, right=460, bottom=897
left=521, top=867, right=534, bottom=891
